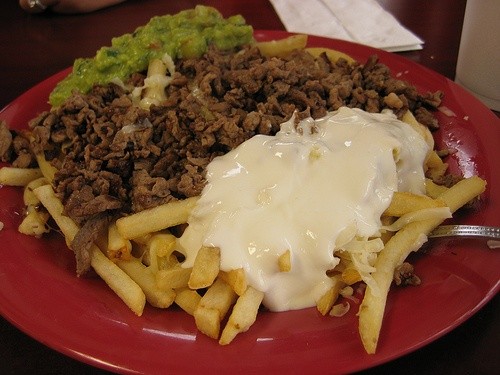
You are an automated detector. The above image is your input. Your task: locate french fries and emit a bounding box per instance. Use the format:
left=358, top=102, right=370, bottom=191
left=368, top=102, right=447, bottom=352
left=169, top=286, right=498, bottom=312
left=0, top=35, right=486, bottom=355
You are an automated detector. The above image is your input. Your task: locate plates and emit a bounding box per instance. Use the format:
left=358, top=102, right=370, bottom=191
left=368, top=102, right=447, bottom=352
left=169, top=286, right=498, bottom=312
left=1, top=28, right=499, bottom=374
